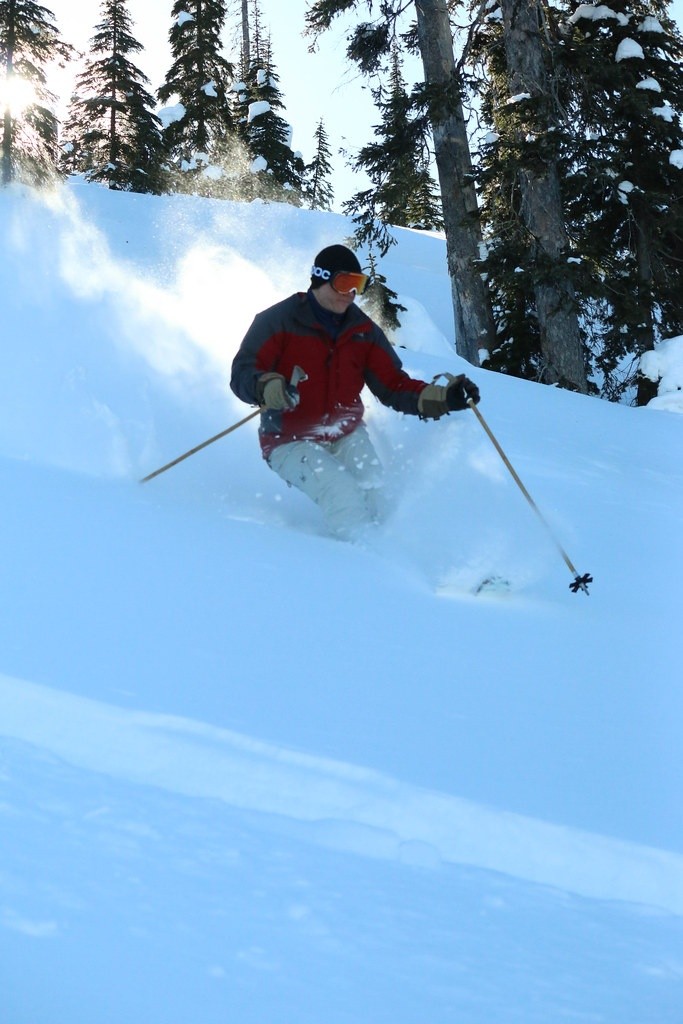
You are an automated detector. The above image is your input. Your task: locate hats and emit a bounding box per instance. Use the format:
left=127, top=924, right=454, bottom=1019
left=311, top=245, right=361, bottom=290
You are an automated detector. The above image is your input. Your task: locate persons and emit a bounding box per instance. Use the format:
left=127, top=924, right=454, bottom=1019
left=230, top=244, right=481, bottom=544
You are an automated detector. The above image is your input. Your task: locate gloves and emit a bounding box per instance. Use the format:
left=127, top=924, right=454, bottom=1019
left=418, top=374, right=479, bottom=422
left=258, top=373, right=300, bottom=409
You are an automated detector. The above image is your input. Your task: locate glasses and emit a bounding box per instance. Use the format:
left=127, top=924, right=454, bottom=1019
left=329, top=271, right=372, bottom=296
